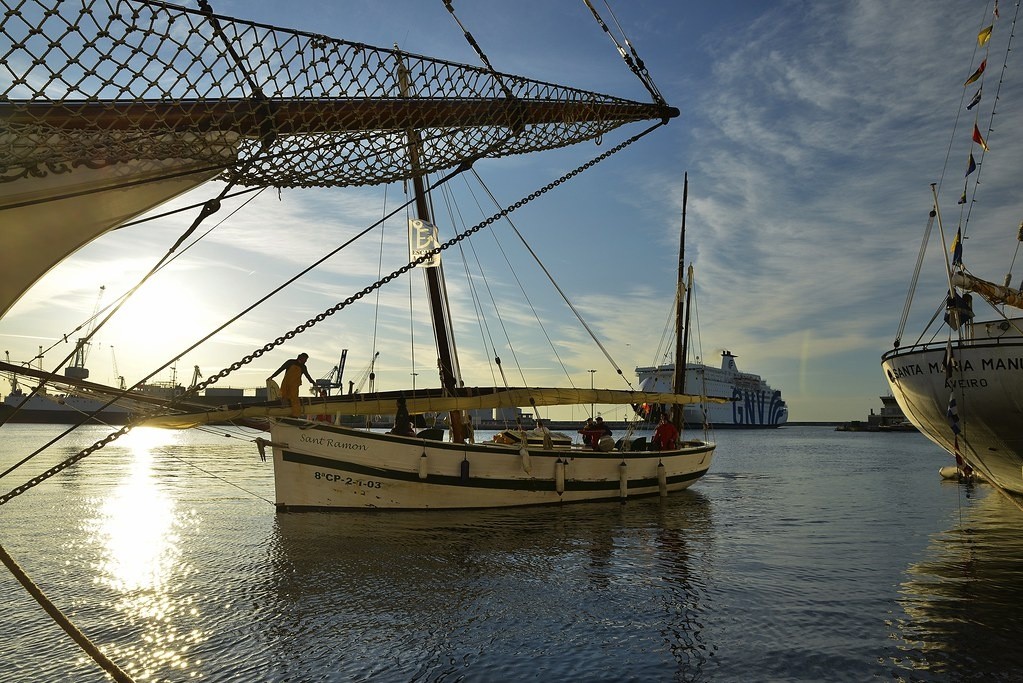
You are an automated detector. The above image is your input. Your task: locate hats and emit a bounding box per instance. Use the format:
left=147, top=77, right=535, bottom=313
left=585, top=418, right=592, bottom=422
left=596, top=417, right=602, bottom=421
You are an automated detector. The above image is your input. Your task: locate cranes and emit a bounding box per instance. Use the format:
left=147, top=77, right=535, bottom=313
left=317, top=349, right=348, bottom=388
left=65, top=286, right=105, bottom=379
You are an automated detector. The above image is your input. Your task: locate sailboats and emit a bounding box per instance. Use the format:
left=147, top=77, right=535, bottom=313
left=270, top=42, right=717, bottom=513
left=881, top=0, right=1023, bottom=498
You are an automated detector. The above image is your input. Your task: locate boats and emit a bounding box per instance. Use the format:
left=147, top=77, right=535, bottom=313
left=1, top=371, right=200, bottom=425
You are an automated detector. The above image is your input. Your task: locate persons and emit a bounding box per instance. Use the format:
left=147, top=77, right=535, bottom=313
left=268, top=353, right=321, bottom=418
left=392, top=398, right=410, bottom=436
left=651, top=417, right=678, bottom=450
left=582, top=417, right=613, bottom=445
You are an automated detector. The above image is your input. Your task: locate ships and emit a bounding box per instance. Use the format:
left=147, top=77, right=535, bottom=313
left=632, top=350, right=789, bottom=429
